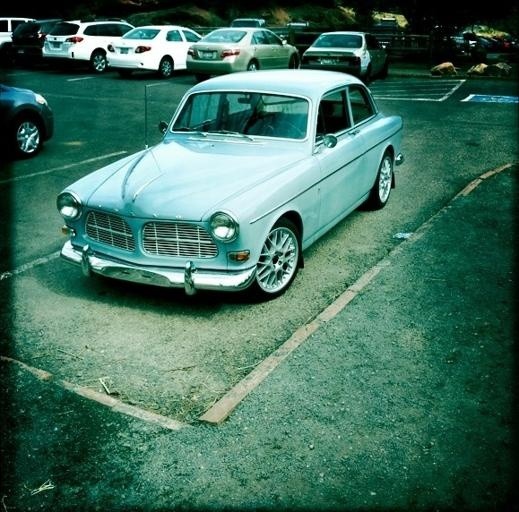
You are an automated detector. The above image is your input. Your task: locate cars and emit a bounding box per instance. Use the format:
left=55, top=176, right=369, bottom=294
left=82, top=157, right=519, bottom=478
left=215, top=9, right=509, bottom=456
left=105, top=24, right=203, bottom=78
left=42, top=17, right=136, bottom=74
left=11, top=18, right=63, bottom=70
left=1, top=80, right=53, bottom=161
left=54, top=66, right=404, bottom=302
left=185, top=25, right=301, bottom=83
left=300, top=29, right=390, bottom=86
